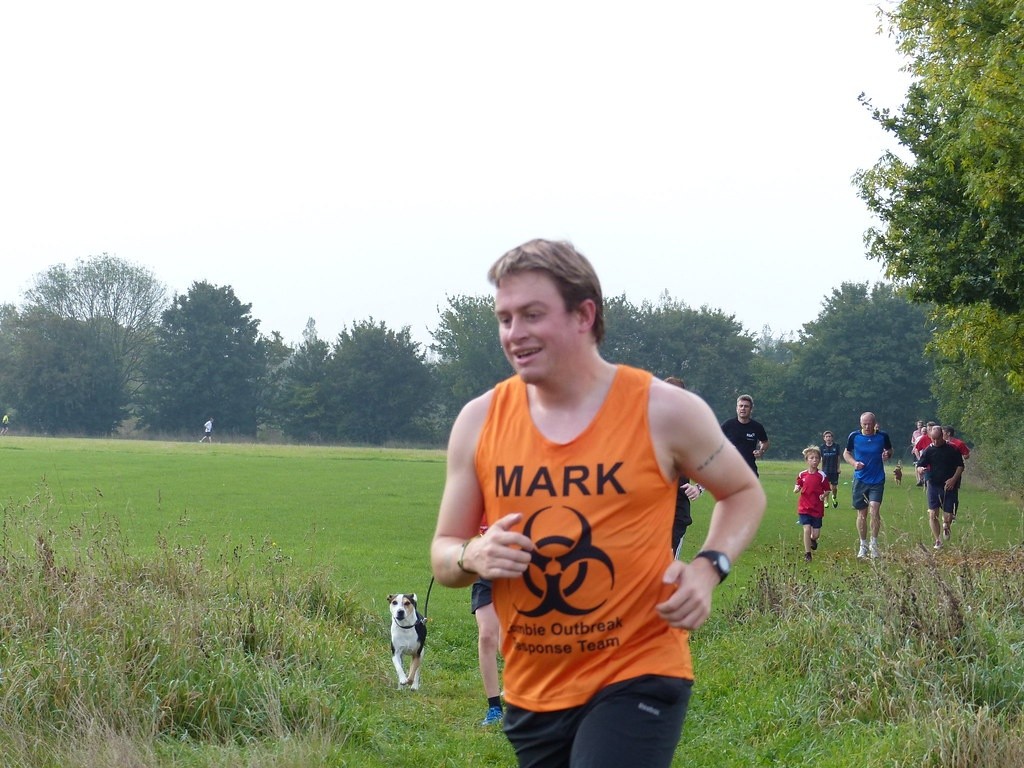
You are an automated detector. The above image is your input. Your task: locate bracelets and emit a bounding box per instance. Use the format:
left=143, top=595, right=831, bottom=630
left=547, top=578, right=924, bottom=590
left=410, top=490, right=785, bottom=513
left=458, top=535, right=484, bottom=574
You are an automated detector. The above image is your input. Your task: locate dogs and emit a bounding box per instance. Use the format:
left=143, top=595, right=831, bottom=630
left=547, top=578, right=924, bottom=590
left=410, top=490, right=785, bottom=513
left=893, top=461, right=902, bottom=486
left=386, top=575, right=435, bottom=692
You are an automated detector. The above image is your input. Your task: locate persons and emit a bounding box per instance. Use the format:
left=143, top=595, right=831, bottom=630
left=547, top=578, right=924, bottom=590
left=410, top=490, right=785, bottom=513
left=818, top=431, right=841, bottom=508
left=0, top=413, right=9, bottom=436
left=911, top=421, right=970, bottom=524
left=875, top=424, right=880, bottom=430
left=794, top=445, right=831, bottom=562
left=916, top=430, right=964, bottom=550
left=199, top=418, right=214, bottom=443
left=892, top=467, right=902, bottom=485
left=472, top=511, right=504, bottom=726
left=661, top=376, right=706, bottom=562
left=720, top=394, right=770, bottom=480
left=429, top=239, right=767, bottom=768
left=843, top=412, right=894, bottom=559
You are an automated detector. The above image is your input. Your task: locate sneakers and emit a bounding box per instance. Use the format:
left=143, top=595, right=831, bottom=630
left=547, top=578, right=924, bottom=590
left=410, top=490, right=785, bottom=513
left=481, top=706, right=504, bottom=726
left=934, top=539, right=943, bottom=549
left=868, top=544, right=881, bottom=558
left=810, top=538, right=818, bottom=550
left=831, top=495, right=838, bottom=508
left=942, top=521, right=952, bottom=541
left=856, top=542, right=869, bottom=559
left=824, top=496, right=829, bottom=509
left=804, top=552, right=812, bottom=562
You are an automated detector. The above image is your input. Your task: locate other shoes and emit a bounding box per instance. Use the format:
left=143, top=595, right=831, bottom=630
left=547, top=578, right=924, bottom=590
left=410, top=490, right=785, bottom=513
left=951, top=514, right=956, bottom=524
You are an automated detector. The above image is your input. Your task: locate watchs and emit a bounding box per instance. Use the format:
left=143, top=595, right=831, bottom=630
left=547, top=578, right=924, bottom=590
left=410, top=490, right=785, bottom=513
left=696, top=484, right=702, bottom=493
left=695, top=551, right=731, bottom=583
